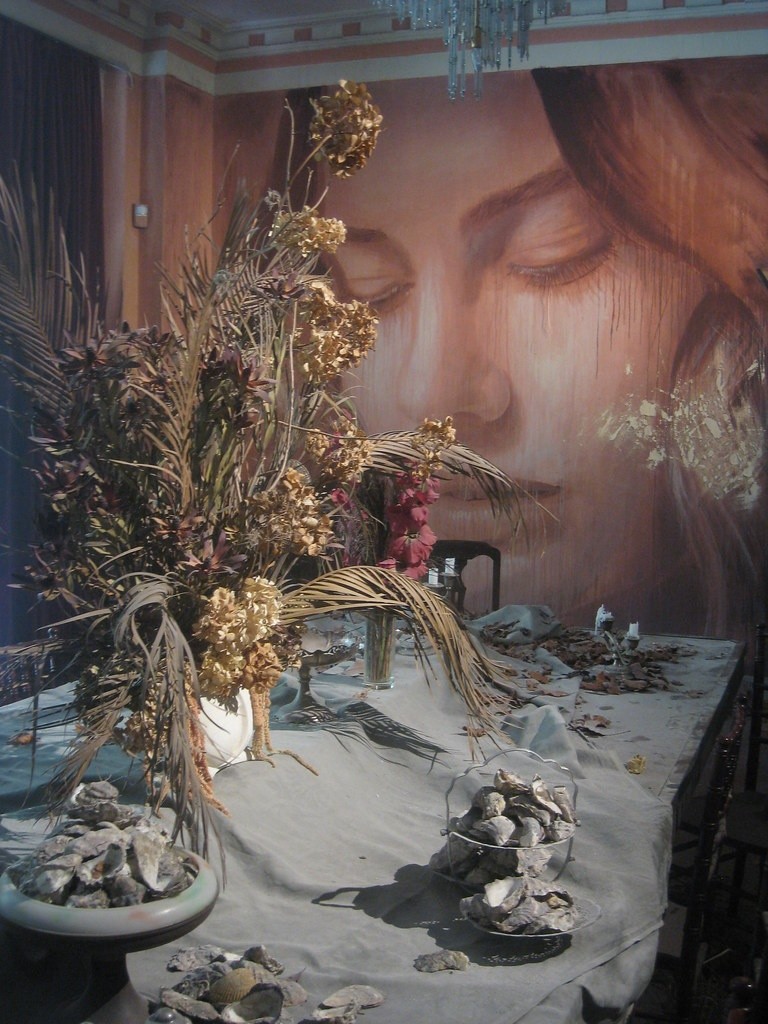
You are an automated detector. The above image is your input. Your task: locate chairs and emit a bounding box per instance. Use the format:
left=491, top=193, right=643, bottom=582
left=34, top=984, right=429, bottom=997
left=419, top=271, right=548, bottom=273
left=370, top=537, right=501, bottom=620
left=669, top=619, right=768, bottom=941
left=633, top=693, right=747, bottom=1020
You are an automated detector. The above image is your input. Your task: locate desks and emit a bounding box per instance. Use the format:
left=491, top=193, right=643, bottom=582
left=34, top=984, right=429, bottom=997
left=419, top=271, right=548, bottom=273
left=1, top=609, right=746, bottom=1024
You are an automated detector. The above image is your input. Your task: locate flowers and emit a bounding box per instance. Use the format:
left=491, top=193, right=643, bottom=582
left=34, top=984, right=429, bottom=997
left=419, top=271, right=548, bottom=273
left=3, top=72, right=554, bottom=878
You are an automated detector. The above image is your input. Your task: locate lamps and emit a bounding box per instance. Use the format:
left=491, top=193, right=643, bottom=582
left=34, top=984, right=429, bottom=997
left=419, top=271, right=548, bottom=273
left=373, top=0, right=569, bottom=102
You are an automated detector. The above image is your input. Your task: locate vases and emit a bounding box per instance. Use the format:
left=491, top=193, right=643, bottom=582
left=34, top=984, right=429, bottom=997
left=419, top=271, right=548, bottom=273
left=178, top=682, right=258, bottom=783
